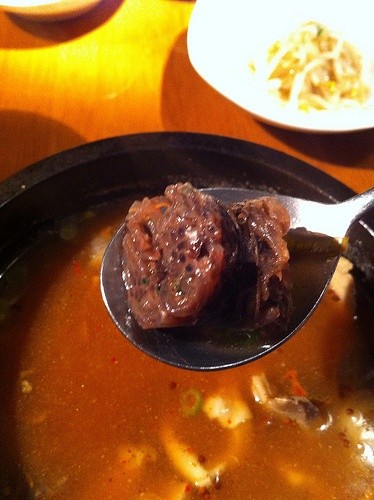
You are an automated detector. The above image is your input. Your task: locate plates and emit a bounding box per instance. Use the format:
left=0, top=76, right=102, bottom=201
left=187, top=0, right=374, bottom=133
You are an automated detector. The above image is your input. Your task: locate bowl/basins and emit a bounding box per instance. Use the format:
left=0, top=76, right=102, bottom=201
left=0, top=0, right=101, bottom=22
left=0, top=131, right=374, bottom=500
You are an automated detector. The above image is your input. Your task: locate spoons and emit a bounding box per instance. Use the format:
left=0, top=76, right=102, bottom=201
left=101, top=182, right=374, bottom=372
